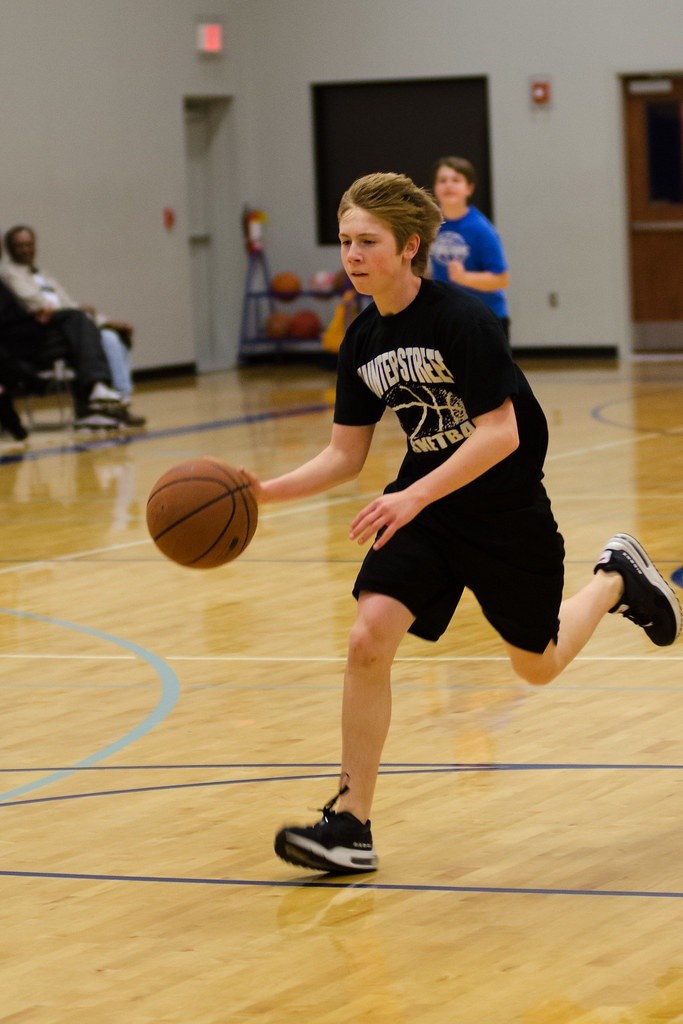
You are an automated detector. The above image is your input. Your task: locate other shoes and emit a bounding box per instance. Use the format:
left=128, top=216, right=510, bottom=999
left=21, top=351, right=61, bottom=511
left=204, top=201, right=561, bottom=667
left=73, top=422, right=126, bottom=433
left=100, top=408, right=145, bottom=427
left=89, top=387, right=129, bottom=411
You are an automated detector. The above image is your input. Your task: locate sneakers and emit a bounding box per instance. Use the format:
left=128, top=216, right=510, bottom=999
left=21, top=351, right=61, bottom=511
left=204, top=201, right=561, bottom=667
left=593, top=534, right=683, bottom=648
left=275, top=772, right=379, bottom=873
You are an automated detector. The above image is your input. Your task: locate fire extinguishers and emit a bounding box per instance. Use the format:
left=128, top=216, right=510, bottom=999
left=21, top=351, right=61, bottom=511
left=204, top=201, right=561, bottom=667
left=242, top=203, right=265, bottom=254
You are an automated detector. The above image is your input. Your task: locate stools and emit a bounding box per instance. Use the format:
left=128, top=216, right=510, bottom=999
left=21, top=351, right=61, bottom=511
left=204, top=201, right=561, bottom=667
left=15, top=356, right=78, bottom=431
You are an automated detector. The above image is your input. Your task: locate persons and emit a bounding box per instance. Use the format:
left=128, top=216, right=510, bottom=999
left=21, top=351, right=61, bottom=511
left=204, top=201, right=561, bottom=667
left=429, top=158, right=511, bottom=344
left=0, top=226, right=146, bottom=431
left=240, top=173, right=683, bottom=870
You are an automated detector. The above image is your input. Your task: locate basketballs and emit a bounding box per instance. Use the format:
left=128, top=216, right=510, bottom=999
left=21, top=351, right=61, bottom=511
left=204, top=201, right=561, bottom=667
left=145, top=458, right=260, bottom=572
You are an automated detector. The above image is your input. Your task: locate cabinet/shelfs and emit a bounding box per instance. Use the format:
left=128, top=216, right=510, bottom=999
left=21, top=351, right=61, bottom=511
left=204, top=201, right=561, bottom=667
left=234, top=253, right=374, bottom=373
